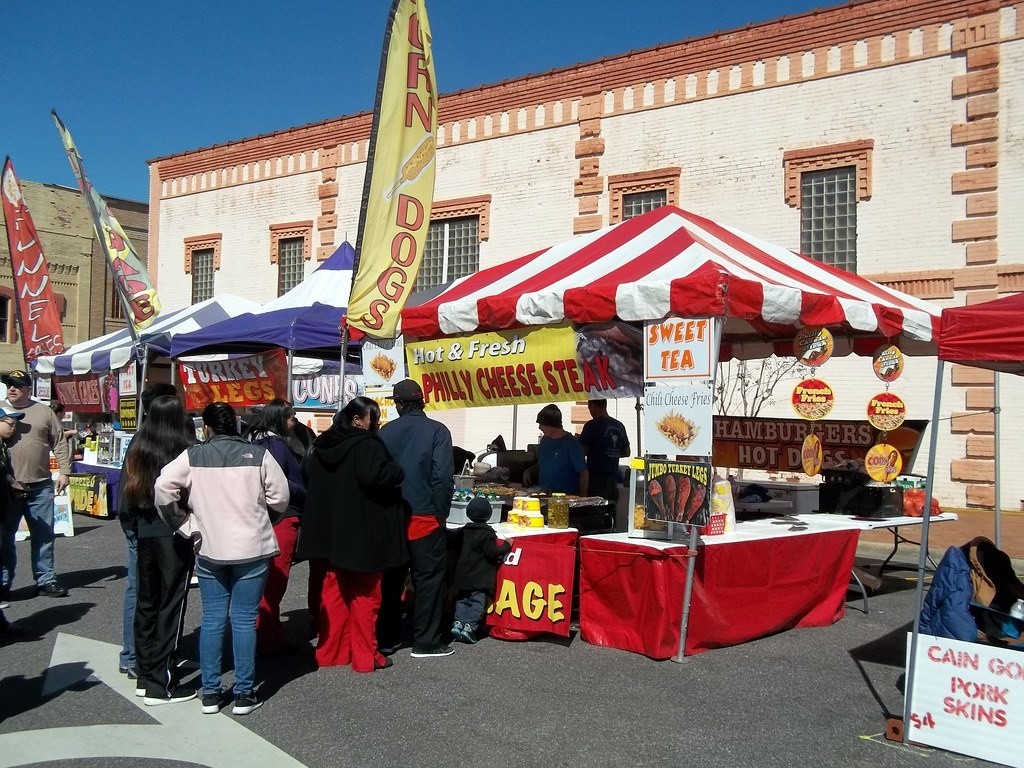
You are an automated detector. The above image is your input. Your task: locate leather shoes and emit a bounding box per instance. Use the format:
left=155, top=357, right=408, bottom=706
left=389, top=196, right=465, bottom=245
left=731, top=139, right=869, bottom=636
left=36, top=581, right=69, bottom=598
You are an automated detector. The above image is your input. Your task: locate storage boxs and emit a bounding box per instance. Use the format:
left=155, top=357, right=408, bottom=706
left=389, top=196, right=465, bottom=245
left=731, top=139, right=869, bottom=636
left=446, top=500, right=505, bottom=524
left=859, top=485, right=904, bottom=519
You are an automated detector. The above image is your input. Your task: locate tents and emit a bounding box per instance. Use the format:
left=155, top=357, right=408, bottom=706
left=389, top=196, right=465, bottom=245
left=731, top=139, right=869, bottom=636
left=166, top=237, right=362, bottom=448
left=28, top=293, right=237, bottom=524
left=337, top=203, right=1006, bottom=667
left=897, top=287, right=1023, bottom=745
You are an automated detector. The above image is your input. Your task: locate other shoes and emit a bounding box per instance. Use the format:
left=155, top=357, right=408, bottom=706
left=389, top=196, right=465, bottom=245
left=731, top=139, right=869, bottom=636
left=262, top=644, right=302, bottom=658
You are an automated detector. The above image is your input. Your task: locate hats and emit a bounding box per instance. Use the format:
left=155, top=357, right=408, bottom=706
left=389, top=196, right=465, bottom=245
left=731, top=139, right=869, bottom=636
left=462, top=497, right=494, bottom=525
left=1, top=369, right=33, bottom=388
left=0, top=406, right=26, bottom=423
left=535, top=405, right=563, bottom=430
left=385, top=378, right=425, bottom=402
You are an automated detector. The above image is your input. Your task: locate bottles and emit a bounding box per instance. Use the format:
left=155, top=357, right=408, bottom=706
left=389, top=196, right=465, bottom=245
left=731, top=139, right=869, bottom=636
left=452, top=488, right=497, bottom=502
left=1009, top=598, right=1024, bottom=621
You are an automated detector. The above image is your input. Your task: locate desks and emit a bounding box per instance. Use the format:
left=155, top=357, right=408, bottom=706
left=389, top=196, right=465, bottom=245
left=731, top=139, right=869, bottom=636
left=577, top=509, right=959, bottom=662
left=75, top=460, right=123, bottom=515
left=445, top=521, right=579, bottom=641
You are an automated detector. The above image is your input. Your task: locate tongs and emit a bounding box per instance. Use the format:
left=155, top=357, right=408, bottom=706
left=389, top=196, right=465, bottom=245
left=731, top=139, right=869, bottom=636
left=461, top=459, right=471, bottom=476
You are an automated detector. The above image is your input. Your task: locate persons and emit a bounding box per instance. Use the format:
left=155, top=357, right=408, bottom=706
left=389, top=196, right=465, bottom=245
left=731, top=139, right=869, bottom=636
left=370, top=379, right=456, bottom=658
left=0, top=407, right=29, bottom=609
left=238, top=398, right=306, bottom=651
left=117, top=382, right=178, bottom=680
left=0, top=609, right=33, bottom=648
left=49, top=403, right=78, bottom=440
left=0, top=370, right=73, bottom=599
left=447, top=495, right=515, bottom=643
left=523, top=404, right=590, bottom=499
left=576, top=399, right=631, bottom=532
left=120, top=395, right=197, bottom=707
left=153, top=401, right=290, bottom=717
left=300, top=395, right=394, bottom=675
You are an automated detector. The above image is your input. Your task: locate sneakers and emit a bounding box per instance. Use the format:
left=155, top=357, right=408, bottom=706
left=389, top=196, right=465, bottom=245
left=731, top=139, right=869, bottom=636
left=378, top=639, right=404, bottom=654
left=233, top=689, right=265, bottom=716
left=460, top=623, right=478, bottom=645
left=144, top=687, right=200, bottom=707
left=370, top=655, right=394, bottom=669
left=410, top=640, right=455, bottom=659
left=450, top=620, right=463, bottom=640
left=199, top=691, right=225, bottom=715
left=134, top=678, right=148, bottom=699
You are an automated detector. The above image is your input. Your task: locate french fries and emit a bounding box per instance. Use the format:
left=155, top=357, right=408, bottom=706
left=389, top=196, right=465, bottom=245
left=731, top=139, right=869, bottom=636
left=656, top=408, right=700, bottom=451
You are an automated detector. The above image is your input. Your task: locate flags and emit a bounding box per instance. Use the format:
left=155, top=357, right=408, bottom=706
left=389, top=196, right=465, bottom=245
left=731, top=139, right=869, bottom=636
left=345, top=0, right=439, bottom=337
left=3, top=156, right=67, bottom=364
left=50, top=109, right=160, bottom=334
